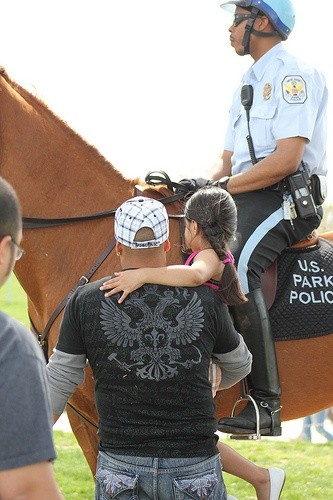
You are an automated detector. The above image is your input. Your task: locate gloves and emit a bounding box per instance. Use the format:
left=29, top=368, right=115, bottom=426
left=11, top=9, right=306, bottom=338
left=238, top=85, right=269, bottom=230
left=174, top=178, right=216, bottom=194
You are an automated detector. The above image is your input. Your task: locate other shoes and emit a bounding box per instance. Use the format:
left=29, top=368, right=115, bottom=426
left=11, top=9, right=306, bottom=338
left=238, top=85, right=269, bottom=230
left=266, top=467, right=286, bottom=500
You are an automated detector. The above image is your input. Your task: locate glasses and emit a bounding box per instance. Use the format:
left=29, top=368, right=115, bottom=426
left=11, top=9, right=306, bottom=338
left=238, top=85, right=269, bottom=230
left=232, top=13, right=261, bottom=27
left=3, top=233, right=25, bottom=262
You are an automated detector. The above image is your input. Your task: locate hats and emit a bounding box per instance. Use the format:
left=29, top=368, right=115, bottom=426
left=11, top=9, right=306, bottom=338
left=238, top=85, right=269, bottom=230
left=113, top=195, right=169, bottom=249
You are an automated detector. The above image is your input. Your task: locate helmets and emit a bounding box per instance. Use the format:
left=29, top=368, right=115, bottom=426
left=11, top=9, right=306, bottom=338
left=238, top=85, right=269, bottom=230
left=235, top=0, right=296, bottom=41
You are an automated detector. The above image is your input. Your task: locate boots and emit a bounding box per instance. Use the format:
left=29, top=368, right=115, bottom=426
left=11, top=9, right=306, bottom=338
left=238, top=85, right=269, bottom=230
left=217, top=286, right=284, bottom=436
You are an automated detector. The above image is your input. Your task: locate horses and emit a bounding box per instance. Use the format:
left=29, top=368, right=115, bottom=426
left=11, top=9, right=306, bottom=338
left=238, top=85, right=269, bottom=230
left=0, top=66, right=333, bottom=481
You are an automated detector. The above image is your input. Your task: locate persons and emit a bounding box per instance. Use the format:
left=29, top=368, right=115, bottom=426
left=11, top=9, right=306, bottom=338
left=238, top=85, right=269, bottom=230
left=47, top=196, right=252, bottom=500
left=207, top=1, right=328, bottom=437
left=100, top=186, right=286, bottom=500
left=0, top=177, right=67, bottom=500
